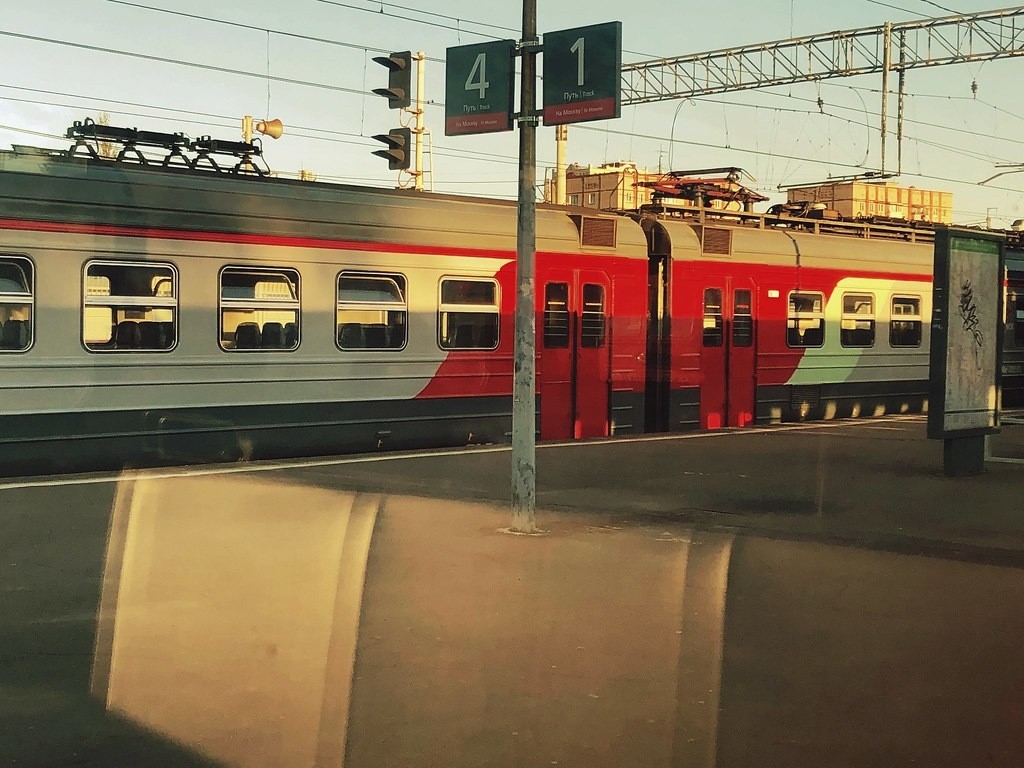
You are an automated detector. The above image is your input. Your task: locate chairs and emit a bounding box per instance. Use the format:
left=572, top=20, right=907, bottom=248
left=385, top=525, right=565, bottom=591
left=339, top=323, right=366, bottom=348
left=117, top=321, right=140, bottom=348
left=479, top=324, right=497, bottom=347
left=260, top=322, right=285, bottom=349
left=892, top=330, right=902, bottom=346
left=787, top=328, right=802, bottom=345
left=284, top=323, right=299, bottom=349
left=367, top=324, right=391, bottom=348
left=3, top=320, right=25, bottom=350
left=138, top=322, right=165, bottom=349
left=802, top=328, right=823, bottom=345
left=234, top=322, right=262, bottom=349
left=842, top=329, right=873, bottom=345
left=454, top=325, right=480, bottom=348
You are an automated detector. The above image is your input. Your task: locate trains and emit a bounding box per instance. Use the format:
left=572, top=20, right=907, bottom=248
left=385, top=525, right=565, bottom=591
left=0, top=148, right=1024, bottom=471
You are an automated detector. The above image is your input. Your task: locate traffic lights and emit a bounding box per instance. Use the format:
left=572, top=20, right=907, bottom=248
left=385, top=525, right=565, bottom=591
left=371, top=52, right=411, bottom=110
left=372, top=128, right=412, bottom=170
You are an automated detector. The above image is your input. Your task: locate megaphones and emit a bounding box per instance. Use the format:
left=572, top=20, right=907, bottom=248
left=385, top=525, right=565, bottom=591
left=256, top=119, right=283, bottom=140
